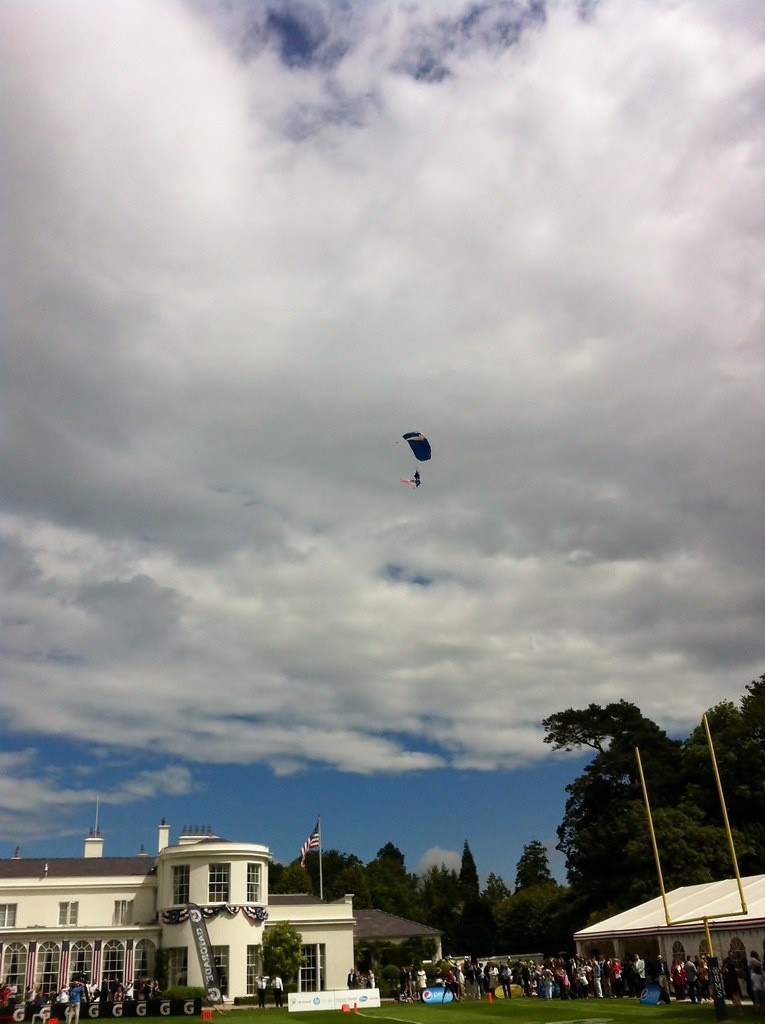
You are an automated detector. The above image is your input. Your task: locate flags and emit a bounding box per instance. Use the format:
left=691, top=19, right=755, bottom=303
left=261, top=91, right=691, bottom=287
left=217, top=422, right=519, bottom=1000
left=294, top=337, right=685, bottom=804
left=301, top=821, right=319, bottom=868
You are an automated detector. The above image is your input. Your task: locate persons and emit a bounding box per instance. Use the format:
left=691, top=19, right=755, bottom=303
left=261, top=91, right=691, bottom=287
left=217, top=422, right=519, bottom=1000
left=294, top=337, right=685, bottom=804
left=0, top=970, right=163, bottom=1024
left=414, top=470, right=421, bottom=488
left=348, top=951, right=765, bottom=1016
left=255, top=975, right=270, bottom=1008
left=271, top=974, right=283, bottom=1008
